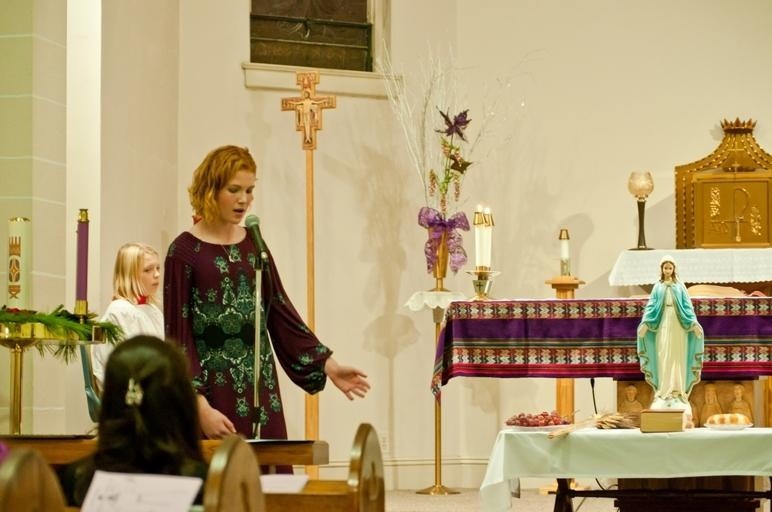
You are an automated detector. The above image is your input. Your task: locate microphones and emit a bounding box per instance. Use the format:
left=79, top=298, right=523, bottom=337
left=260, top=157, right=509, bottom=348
left=245, top=214, right=268, bottom=265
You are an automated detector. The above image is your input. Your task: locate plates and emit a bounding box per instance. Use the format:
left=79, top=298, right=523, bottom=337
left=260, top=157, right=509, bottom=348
left=505, top=424, right=570, bottom=434
left=703, top=422, right=752, bottom=431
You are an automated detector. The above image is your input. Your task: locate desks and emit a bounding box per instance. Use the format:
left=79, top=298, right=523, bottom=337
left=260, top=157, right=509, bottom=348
left=607, top=246, right=772, bottom=294
left=480, top=420, right=772, bottom=512
left=441, top=294, right=770, bottom=386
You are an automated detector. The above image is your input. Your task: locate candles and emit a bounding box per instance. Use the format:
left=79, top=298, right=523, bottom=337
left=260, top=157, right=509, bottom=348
left=474, top=205, right=485, bottom=266
left=483, top=208, right=495, bottom=266
left=559, top=229, right=569, bottom=263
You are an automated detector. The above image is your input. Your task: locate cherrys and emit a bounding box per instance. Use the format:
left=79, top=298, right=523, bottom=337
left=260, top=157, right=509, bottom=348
left=506, top=410, right=572, bottom=426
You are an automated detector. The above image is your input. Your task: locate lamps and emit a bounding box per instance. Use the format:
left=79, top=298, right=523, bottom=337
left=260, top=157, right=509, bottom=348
left=626, top=171, right=655, bottom=251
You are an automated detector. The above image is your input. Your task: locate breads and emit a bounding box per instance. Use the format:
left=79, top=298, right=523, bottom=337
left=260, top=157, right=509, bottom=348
left=706, top=413, right=751, bottom=426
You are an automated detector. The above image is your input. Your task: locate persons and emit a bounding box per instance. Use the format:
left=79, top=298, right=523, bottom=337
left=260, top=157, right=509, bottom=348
left=91, top=242, right=165, bottom=398
left=72, top=335, right=209, bottom=506
left=699, top=383, right=722, bottom=427
left=637, top=254, right=704, bottom=403
left=163, top=146, right=370, bottom=473
left=617, top=385, right=643, bottom=427
left=727, top=384, right=753, bottom=422
left=287, top=91, right=329, bottom=144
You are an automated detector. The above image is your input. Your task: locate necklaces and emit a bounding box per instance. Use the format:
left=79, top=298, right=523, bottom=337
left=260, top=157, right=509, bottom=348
left=222, top=244, right=234, bottom=263
